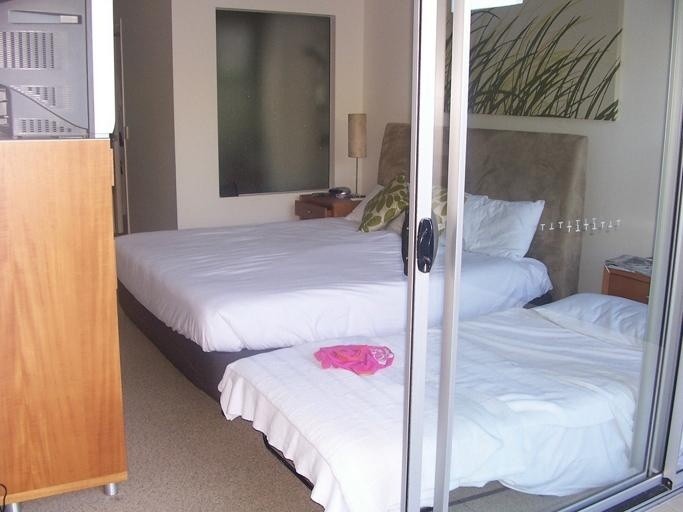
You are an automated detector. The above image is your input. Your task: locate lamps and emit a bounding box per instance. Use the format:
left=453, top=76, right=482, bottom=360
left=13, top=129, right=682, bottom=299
left=346, top=111, right=369, bottom=198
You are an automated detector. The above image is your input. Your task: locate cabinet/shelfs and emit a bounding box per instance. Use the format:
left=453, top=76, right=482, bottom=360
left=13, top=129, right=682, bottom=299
left=0, top=136, right=124, bottom=512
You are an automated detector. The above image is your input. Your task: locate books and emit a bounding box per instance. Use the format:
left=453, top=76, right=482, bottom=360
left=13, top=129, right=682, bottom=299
left=604, top=253, right=653, bottom=279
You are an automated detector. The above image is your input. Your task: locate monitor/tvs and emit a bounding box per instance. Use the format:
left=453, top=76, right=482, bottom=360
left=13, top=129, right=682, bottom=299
left=0, top=0, right=116, bottom=141
